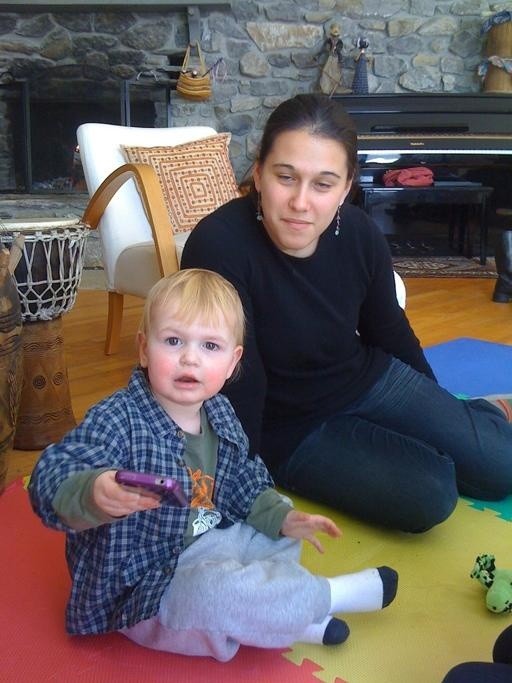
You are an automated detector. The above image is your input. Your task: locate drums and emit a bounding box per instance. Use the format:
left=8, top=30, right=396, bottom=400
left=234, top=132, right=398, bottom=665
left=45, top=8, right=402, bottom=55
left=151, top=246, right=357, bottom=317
left=0, top=217, right=92, bottom=452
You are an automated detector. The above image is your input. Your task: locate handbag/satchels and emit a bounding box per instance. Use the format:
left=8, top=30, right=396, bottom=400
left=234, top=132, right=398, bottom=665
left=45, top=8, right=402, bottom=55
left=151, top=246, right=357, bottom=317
left=176, top=39, right=212, bottom=101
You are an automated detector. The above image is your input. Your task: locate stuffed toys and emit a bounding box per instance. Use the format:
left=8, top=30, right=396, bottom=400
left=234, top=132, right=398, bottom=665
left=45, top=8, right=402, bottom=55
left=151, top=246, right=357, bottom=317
left=470, top=551, right=511, bottom=614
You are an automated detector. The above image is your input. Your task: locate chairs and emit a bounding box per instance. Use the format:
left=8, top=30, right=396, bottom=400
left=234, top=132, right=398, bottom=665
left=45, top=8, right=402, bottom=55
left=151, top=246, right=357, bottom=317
left=74, top=121, right=242, bottom=355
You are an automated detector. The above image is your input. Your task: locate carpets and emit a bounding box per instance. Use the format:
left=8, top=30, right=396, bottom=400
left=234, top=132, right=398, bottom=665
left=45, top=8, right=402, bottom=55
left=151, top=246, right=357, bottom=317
left=1, top=336, right=512, bottom=683
left=383, top=234, right=501, bottom=280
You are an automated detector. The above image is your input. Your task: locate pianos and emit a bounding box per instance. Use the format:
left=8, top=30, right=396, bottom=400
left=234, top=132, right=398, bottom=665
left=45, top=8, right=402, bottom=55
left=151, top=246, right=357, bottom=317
left=323, top=93, right=512, bottom=268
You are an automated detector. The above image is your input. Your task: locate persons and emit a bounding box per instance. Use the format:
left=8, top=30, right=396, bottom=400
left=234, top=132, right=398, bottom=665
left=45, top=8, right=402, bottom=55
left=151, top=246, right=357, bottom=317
left=350, top=35, right=370, bottom=96
left=28, top=270, right=398, bottom=662
left=180, top=93, right=512, bottom=533
left=314, top=23, right=344, bottom=96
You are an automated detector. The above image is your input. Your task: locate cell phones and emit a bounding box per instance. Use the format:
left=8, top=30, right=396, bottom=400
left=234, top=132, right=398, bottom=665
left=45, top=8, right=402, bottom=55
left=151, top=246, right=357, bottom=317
left=115, top=468, right=189, bottom=516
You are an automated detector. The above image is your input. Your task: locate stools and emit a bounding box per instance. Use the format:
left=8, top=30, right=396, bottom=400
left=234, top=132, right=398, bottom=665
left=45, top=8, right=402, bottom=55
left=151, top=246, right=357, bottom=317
left=357, top=175, right=498, bottom=267
